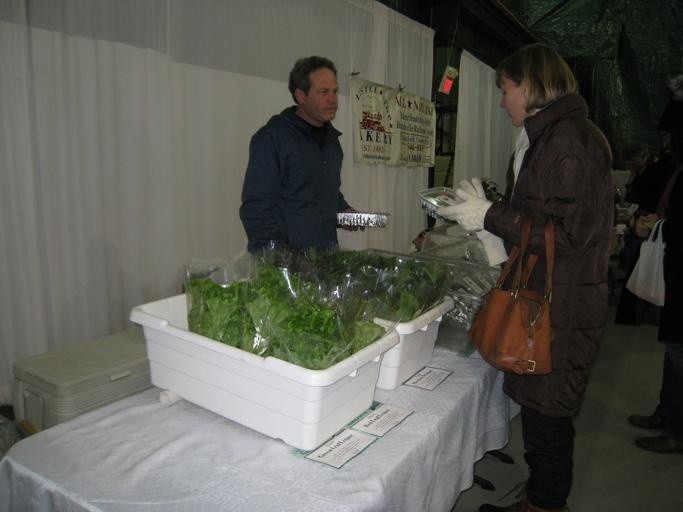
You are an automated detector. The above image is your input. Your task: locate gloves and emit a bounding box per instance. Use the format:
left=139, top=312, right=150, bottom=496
left=453, top=173, right=510, bottom=268
left=436, top=188, right=494, bottom=233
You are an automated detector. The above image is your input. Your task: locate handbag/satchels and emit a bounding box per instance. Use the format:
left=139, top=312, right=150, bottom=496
left=467, top=284, right=555, bottom=377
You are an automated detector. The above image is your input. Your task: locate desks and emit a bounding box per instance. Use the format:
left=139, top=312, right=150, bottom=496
left=0, top=347, right=516, bottom=510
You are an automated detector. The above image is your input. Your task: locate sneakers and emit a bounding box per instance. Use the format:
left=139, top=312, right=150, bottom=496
left=476, top=497, right=571, bottom=512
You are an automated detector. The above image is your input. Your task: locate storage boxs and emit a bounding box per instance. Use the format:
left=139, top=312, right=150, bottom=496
left=374, top=293, right=455, bottom=392
left=129, top=295, right=402, bottom=452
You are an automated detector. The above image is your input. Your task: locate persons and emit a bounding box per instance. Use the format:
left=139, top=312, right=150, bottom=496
left=612, top=73, right=682, bottom=454
left=433, top=42, right=615, bottom=512
left=238, top=55, right=365, bottom=263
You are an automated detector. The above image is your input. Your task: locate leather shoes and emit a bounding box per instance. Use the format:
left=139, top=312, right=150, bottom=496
left=633, top=428, right=683, bottom=454
left=627, top=409, right=669, bottom=430
